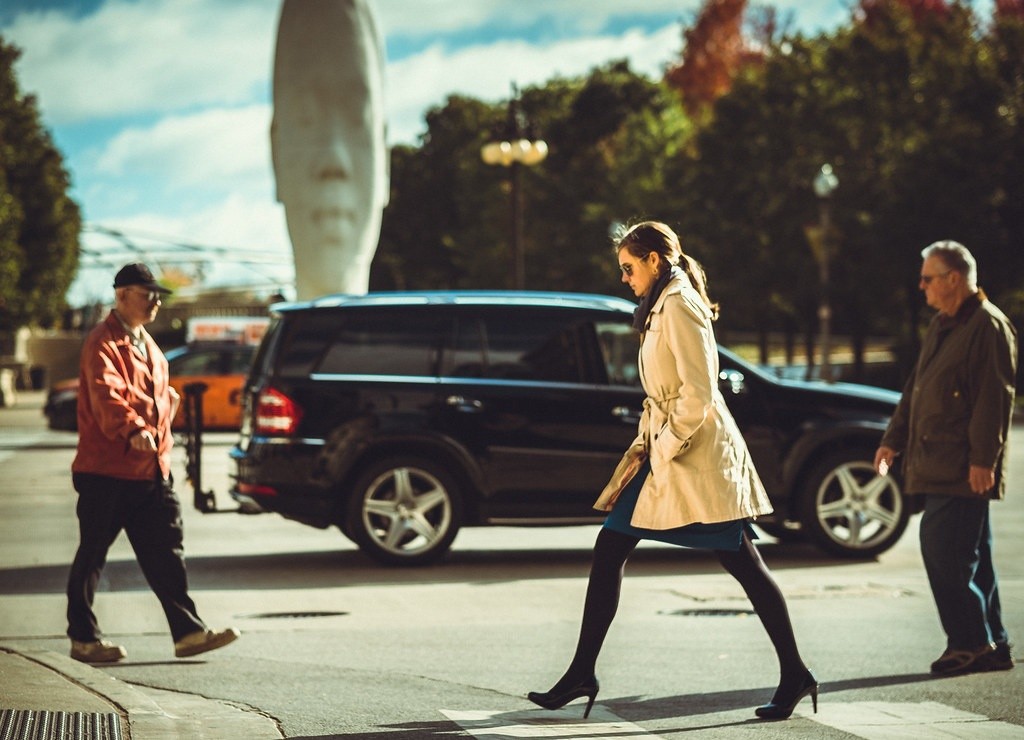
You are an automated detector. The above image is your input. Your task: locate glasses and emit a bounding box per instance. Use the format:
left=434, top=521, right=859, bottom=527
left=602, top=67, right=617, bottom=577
left=122, top=289, right=161, bottom=301
left=920, top=270, right=955, bottom=282
left=620, top=253, right=649, bottom=276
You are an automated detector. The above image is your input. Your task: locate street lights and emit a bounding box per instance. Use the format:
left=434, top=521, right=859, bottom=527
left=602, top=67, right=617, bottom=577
left=479, top=136, right=548, bottom=293
left=811, top=160, right=840, bottom=382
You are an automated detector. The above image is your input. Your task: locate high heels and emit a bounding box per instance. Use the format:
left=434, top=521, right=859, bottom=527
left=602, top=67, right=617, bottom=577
left=528, top=678, right=600, bottom=718
left=756, top=670, right=817, bottom=719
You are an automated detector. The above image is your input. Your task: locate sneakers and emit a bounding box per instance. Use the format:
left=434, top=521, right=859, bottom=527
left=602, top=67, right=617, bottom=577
left=966, top=640, right=1014, bottom=670
left=71, top=642, right=126, bottom=662
left=932, top=641, right=997, bottom=672
left=177, top=628, right=238, bottom=656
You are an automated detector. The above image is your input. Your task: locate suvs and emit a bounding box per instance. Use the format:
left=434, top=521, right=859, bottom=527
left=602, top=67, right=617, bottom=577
left=226, top=291, right=926, bottom=566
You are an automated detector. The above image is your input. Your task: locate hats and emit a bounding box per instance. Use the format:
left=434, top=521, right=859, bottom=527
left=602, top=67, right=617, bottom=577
left=113, top=262, right=173, bottom=294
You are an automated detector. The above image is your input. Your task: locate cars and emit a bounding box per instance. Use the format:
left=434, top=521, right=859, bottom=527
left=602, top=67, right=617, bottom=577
left=45, top=340, right=260, bottom=435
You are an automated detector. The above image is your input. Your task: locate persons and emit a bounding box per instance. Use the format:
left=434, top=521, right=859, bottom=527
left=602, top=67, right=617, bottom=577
left=66, top=265, right=238, bottom=661
left=873, top=242, right=1019, bottom=671
left=269, top=0, right=390, bottom=296
left=526, top=220, right=819, bottom=720
